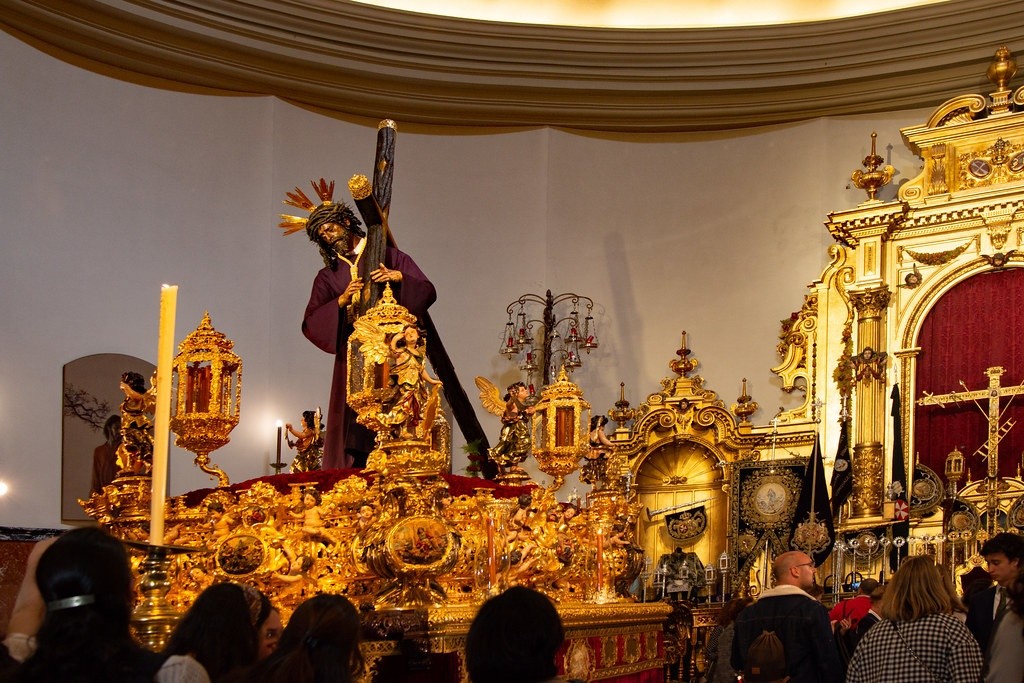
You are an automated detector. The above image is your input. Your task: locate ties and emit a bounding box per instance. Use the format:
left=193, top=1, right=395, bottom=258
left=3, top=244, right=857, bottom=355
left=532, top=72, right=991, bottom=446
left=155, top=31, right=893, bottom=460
left=995, top=588, right=1006, bottom=618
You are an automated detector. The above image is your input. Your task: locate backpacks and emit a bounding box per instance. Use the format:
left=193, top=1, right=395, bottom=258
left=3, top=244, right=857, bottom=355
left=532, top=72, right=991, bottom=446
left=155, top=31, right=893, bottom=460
left=743, top=596, right=812, bottom=682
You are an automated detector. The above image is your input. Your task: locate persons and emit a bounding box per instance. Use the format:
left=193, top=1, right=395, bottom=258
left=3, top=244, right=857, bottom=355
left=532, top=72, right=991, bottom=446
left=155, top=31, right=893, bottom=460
left=286, top=410, right=325, bottom=474
left=271, top=488, right=380, bottom=583
left=301, top=201, right=437, bottom=469
left=90, top=414, right=123, bottom=496
left=605, top=524, right=629, bottom=549
left=189, top=502, right=234, bottom=589
left=729, top=532, right=1024, bottom=683
left=464, top=586, right=565, bottom=683
left=116, top=371, right=155, bottom=474
left=0, top=526, right=362, bottom=683
left=580, top=415, right=615, bottom=490
left=390, top=325, right=443, bottom=442
left=494, top=382, right=534, bottom=464
left=508, top=494, right=578, bottom=563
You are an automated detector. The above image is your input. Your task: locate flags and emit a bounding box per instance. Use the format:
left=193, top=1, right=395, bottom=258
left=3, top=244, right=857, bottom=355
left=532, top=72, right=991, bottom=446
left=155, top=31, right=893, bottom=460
left=889, top=384, right=909, bottom=571
left=831, top=422, right=852, bottom=505
left=788, top=437, right=835, bottom=566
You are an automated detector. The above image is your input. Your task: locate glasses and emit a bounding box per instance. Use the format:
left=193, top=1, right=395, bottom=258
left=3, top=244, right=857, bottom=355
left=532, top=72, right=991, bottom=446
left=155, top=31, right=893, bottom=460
left=794, top=561, right=815, bottom=567
